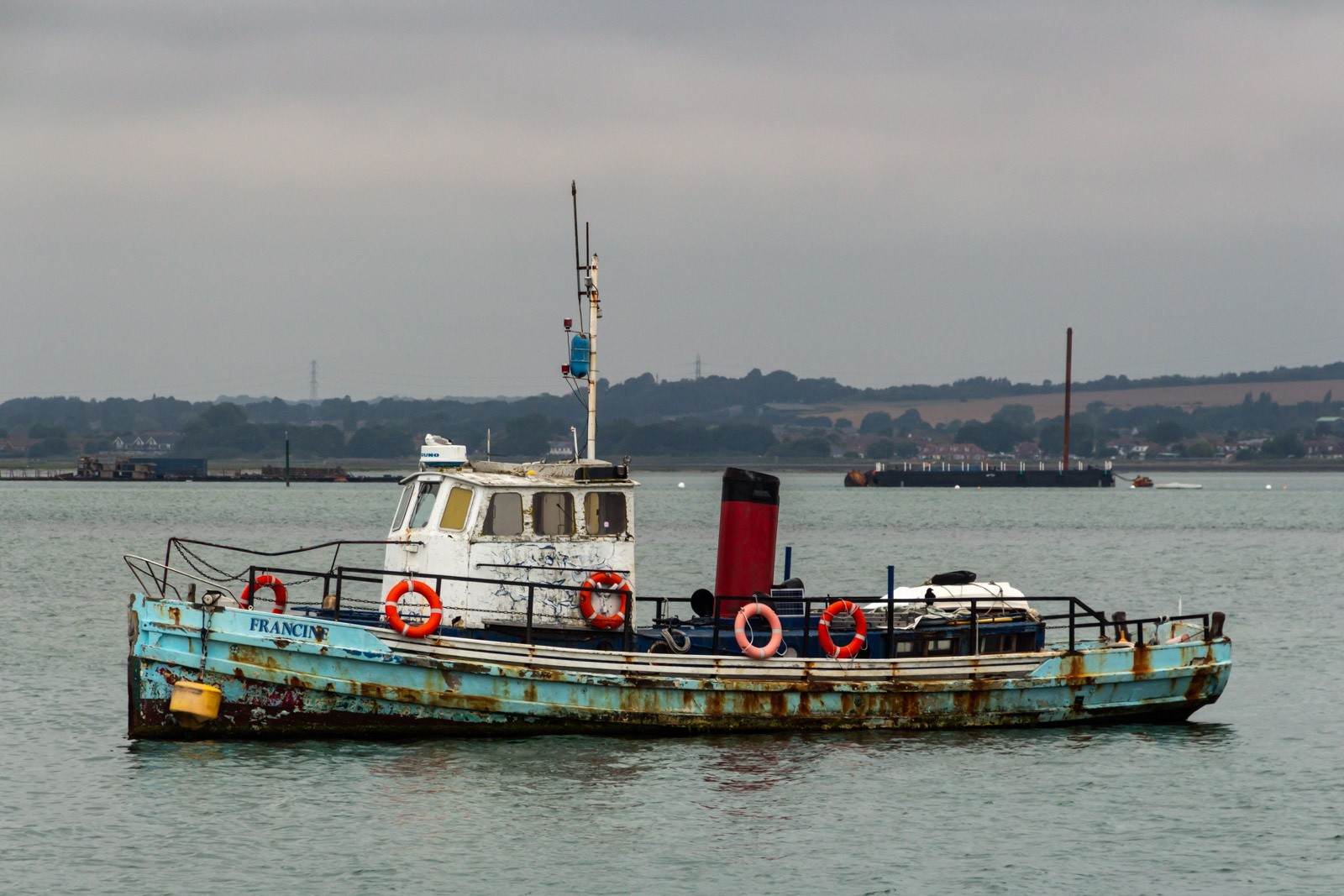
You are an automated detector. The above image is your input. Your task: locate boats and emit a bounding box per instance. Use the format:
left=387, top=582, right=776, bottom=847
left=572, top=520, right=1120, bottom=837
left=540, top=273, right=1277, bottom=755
left=118, top=172, right=1237, bottom=750
left=1133, top=474, right=1154, bottom=488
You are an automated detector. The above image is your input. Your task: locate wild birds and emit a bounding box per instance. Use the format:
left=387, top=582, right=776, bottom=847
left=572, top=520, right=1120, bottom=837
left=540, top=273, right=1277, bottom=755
left=924, top=588, right=937, bottom=607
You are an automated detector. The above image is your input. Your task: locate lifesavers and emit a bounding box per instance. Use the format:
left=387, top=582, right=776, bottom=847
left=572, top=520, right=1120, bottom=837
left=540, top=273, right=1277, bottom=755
left=819, top=599, right=868, bottom=661
left=387, top=580, right=441, bottom=637
left=580, top=572, right=632, bottom=630
left=239, top=574, right=287, bottom=615
left=734, top=603, right=784, bottom=659
